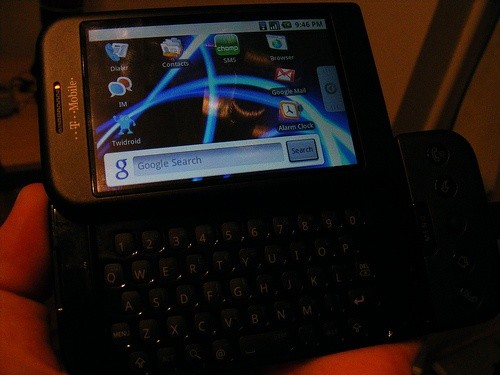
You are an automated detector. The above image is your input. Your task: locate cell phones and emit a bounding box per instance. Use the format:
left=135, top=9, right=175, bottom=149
left=37, top=2, right=489, bottom=375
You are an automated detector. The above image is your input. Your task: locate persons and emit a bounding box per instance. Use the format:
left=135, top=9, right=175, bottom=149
left=0, top=182, right=427, bottom=375
